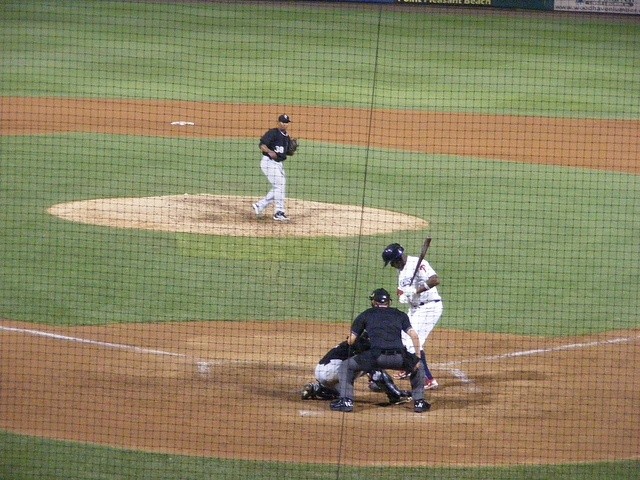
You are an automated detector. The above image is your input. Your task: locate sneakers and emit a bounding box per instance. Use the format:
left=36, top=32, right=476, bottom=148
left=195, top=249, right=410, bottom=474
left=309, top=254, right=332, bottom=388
left=330, top=399, right=353, bottom=411
left=273, top=211, right=291, bottom=221
left=393, top=370, right=410, bottom=379
left=251, top=203, right=259, bottom=215
left=424, top=379, right=439, bottom=390
left=390, top=394, right=413, bottom=405
left=302, top=384, right=313, bottom=399
left=414, top=400, right=430, bottom=412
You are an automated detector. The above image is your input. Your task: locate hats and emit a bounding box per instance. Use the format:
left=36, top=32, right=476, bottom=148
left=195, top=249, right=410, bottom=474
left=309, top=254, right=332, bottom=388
left=279, top=115, right=291, bottom=123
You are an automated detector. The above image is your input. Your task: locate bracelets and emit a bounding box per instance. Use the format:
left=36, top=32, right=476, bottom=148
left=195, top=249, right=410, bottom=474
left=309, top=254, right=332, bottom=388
left=423, top=282, right=430, bottom=290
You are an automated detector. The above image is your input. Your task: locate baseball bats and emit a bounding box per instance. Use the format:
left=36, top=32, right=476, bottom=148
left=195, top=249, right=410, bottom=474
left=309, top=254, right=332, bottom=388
left=410, top=237, right=432, bottom=285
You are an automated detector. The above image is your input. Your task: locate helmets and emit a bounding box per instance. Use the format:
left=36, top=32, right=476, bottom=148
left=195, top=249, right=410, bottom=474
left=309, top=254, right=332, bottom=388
left=369, top=288, right=392, bottom=307
left=382, top=243, right=404, bottom=269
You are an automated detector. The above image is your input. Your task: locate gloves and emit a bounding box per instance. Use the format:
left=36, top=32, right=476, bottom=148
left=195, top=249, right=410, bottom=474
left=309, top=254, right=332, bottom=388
left=399, top=294, right=409, bottom=304
left=403, top=285, right=417, bottom=296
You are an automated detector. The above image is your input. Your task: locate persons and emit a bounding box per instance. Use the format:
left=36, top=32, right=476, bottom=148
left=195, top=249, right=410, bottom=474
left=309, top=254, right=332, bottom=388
left=382, top=243, right=443, bottom=389
left=252, top=114, right=297, bottom=221
left=301, top=332, right=413, bottom=404
left=330, top=289, right=430, bottom=412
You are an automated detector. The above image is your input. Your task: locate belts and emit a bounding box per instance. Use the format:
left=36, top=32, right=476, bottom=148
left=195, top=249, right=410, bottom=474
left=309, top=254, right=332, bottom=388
left=420, top=300, right=440, bottom=305
left=382, top=349, right=400, bottom=355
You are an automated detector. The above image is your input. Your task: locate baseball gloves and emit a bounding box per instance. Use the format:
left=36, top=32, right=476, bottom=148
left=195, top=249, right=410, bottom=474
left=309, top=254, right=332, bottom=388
left=289, top=137, right=297, bottom=152
